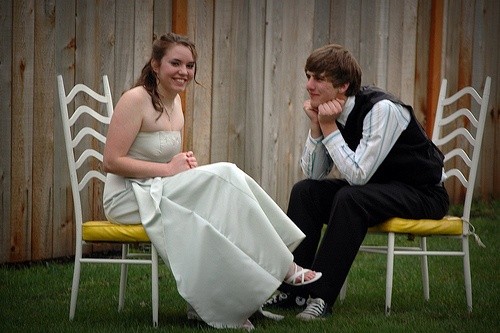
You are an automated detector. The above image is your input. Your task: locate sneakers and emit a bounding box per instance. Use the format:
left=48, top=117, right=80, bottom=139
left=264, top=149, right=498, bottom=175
left=262, top=292, right=333, bottom=320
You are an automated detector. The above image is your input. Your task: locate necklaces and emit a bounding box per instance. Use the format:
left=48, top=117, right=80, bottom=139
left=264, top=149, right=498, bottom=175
left=159, top=95, right=174, bottom=121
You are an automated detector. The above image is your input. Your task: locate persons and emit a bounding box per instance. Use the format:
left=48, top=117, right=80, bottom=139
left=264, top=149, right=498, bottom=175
left=259, top=45, right=450, bottom=322
left=103, top=32, right=323, bottom=331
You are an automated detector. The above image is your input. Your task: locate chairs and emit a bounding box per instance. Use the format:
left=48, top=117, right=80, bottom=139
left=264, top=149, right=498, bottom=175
left=57, top=74, right=158, bottom=330
left=340, top=76, right=491, bottom=317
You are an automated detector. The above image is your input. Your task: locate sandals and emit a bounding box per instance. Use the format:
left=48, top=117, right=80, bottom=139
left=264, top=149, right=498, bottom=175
left=284, top=262, right=324, bottom=286
